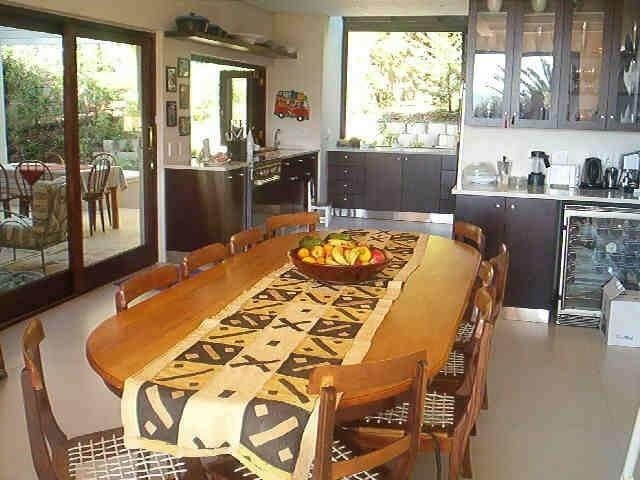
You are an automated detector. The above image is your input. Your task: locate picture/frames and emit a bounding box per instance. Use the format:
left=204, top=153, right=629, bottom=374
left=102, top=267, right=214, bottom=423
left=162, top=57, right=190, bottom=137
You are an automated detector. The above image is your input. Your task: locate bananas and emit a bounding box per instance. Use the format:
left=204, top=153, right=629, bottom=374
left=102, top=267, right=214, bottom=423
left=326, top=247, right=361, bottom=265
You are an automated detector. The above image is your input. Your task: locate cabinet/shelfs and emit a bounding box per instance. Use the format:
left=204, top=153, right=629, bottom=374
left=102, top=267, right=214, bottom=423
left=454, top=197, right=559, bottom=325
left=464, top=0, right=640, bottom=129
left=166, top=153, right=319, bottom=252
left=327, top=147, right=458, bottom=212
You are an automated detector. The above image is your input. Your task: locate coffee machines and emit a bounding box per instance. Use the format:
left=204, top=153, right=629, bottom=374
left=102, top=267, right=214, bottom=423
left=526, top=151, right=552, bottom=186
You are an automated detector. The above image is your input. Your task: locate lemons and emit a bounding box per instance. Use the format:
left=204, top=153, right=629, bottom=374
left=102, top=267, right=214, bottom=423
left=359, top=245, right=372, bottom=262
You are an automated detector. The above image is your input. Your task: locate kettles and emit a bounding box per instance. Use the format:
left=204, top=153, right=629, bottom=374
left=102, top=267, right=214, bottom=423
left=580, top=157, right=603, bottom=190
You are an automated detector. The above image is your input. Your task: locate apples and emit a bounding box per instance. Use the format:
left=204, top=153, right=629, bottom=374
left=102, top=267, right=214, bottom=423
left=372, top=249, right=385, bottom=261
left=298, top=245, right=325, bottom=264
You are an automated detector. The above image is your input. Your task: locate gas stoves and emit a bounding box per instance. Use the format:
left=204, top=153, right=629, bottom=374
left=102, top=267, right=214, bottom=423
left=241, top=145, right=283, bottom=164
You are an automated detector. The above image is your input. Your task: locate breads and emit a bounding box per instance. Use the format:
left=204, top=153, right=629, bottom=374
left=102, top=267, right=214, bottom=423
left=208, top=158, right=216, bottom=163
left=214, top=153, right=228, bottom=162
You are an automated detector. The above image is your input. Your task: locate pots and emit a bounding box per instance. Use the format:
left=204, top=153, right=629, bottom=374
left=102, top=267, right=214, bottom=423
left=174, top=9, right=209, bottom=34
left=207, top=22, right=228, bottom=40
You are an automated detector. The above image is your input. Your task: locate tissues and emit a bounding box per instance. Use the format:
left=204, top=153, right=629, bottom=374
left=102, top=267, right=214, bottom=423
left=253, top=143, right=280, bottom=161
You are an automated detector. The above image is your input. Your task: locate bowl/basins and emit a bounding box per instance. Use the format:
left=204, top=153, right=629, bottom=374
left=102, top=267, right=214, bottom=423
left=286, top=245, right=390, bottom=285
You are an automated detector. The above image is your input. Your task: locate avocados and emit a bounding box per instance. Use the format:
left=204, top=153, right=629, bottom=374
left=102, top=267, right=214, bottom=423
left=324, top=232, right=352, bottom=241
left=299, top=236, right=327, bottom=249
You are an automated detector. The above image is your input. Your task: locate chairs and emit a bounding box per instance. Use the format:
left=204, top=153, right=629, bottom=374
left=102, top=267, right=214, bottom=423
left=204, top=347, right=430, bottom=480
left=435, top=289, right=493, bottom=440
left=458, top=242, right=509, bottom=417
left=267, top=211, right=318, bottom=241
left=114, top=265, right=178, bottom=313
left=184, top=242, right=228, bottom=277
left=341, top=320, right=492, bottom=479
left=453, top=222, right=486, bottom=255
left=18, top=317, right=204, bottom=480
left=229, top=224, right=261, bottom=255
left=1, top=151, right=125, bottom=274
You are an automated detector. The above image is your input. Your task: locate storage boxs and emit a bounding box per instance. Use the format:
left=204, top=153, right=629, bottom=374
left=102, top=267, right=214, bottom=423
left=601, top=278, right=640, bottom=347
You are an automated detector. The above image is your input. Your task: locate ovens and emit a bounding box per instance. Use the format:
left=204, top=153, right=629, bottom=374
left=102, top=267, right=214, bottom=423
left=249, top=161, right=282, bottom=248
